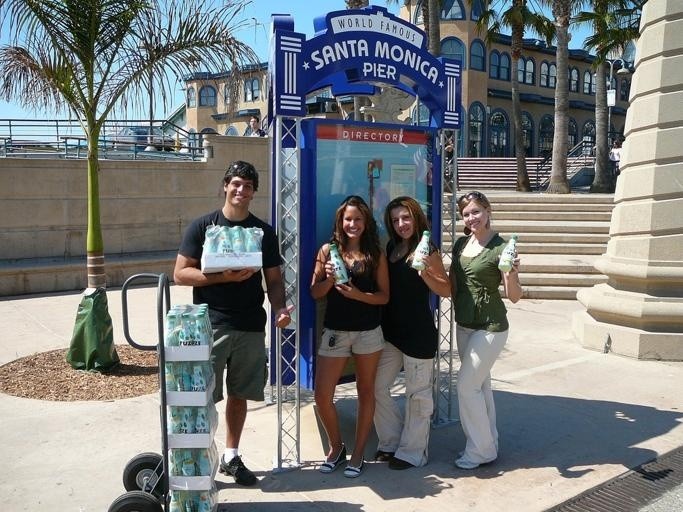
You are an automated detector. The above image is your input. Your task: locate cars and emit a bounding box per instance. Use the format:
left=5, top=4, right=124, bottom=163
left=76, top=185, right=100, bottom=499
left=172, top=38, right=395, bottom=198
left=115, top=124, right=177, bottom=151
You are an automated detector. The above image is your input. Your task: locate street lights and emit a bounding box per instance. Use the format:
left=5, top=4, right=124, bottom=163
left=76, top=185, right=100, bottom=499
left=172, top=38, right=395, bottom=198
left=606, top=57, right=631, bottom=145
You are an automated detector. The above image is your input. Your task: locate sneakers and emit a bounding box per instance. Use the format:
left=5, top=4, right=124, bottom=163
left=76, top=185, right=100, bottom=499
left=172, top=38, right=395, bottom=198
left=374, top=450, right=395, bottom=461
left=318, top=442, right=348, bottom=473
left=454, top=455, right=480, bottom=469
left=218, top=453, right=256, bottom=486
left=343, top=454, right=364, bottom=478
left=387, top=457, right=413, bottom=469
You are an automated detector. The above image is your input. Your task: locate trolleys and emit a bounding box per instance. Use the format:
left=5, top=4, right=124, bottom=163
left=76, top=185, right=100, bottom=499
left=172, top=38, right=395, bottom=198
left=108, top=271, right=173, bottom=512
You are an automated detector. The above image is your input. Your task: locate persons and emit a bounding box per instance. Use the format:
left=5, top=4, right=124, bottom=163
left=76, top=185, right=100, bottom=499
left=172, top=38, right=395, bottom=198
left=445, top=135, right=461, bottom=191
left=609, top=140, right=621, bottom=177
left=173, top=161, right=295, bottom=486
left=371, top=196, right=452, bottom=470
left=242, top=115, right=265, bottom=137
left=309, top=195, right=392, bottom=478
left=448, top=192, right=523, bottom=470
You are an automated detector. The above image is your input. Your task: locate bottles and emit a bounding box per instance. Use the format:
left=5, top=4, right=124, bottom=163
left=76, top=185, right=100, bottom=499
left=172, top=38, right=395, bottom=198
left=168, top=400, right=217, bottom=434
left=201, top=224, right=263, bottom=253
left=165, top=357, right=213, bottom=391
left=168, top=440, right=218, bottom=476
left=412, top=231, right=432, bottom=272
left=498, top=233, right=519, bottom=273
left=170, top=482, right=217, bottom=512
left=166, top=304, right=213, bottom=345
left=329, top=244, right=348, bottom=286
